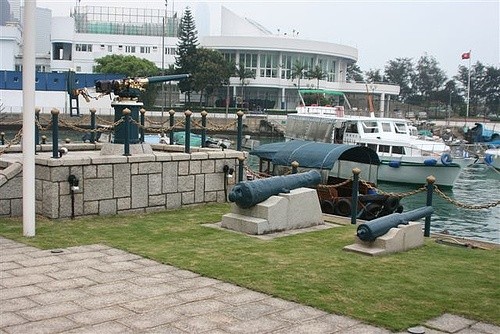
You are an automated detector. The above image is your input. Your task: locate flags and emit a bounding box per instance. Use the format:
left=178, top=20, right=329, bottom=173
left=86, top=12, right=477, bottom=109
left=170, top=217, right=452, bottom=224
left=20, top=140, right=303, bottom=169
left=461, top=50, right=472, bottom=58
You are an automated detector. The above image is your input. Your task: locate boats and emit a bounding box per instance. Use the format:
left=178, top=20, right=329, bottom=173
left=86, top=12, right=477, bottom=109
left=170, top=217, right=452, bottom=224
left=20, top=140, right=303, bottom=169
left=250, top=89, right=499, bottom=221
left=158, top=131, right=231, bottom=151
